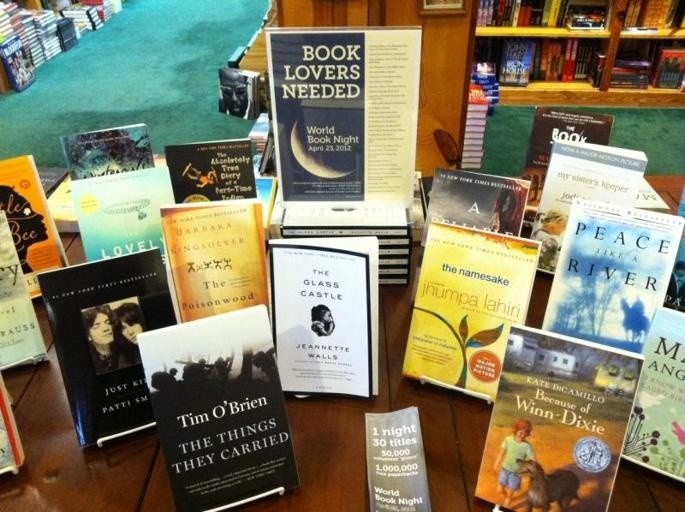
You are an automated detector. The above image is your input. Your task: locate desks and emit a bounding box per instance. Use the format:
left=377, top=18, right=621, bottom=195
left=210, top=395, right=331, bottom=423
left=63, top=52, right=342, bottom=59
left=0, top=176, right=685, bottom=512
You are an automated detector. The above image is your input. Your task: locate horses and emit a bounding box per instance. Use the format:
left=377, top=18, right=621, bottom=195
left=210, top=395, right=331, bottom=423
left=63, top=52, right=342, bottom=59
left=620, top=297, right=651, bottom=345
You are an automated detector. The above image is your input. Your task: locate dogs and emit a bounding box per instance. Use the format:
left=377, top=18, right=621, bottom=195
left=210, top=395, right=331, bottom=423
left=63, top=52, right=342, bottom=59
left=513, top=458, right=582, bottom=512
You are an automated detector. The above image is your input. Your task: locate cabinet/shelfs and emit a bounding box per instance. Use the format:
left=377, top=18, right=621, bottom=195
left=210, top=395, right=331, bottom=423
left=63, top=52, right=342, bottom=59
left=467, top=0, right=685, bottom=106
left=0, top=1, right=45, bottom=93
left=275, top=0, right=480, bottom=177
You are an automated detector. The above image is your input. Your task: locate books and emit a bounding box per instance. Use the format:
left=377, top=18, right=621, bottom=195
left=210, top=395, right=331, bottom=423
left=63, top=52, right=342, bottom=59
left=262, top=8, right=271, bottom=26
left=218, top=67, right=262, bottom=121
left=228, top=47, right=248, bottom=69
left=0, top=0, right=122, bottom=94
left=265, top=25, right=422, bottom=208
left=247, top=29, right=260, bottom=49
left=619, top=307, right=685, bottom=485
left=0, top=368, right=26, bottom=476
left=36, top=246, right=183, bottom=451
left=135, top=303, right=301, bottom=511
left=465, top=2, right=684, bottom=122
left=474, top=324, right=644, bottom=510
